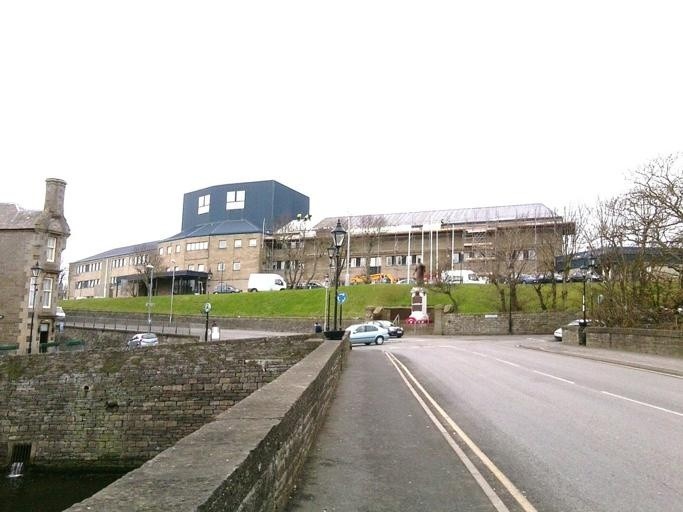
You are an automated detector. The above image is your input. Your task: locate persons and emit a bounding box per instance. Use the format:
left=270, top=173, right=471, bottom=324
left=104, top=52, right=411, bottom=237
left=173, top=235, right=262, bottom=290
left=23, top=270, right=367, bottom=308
left=413, top=258, right=425, bottom=287
left=314, top=323, right=322, bottom=333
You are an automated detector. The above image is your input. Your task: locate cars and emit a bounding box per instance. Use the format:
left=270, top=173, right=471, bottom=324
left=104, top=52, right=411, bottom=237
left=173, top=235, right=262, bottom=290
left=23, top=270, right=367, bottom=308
left=554, top=319, right=605, bottom=341
left=127, top=333, right=159, bottom=347
left=56, top=307, right=66, bottom=317
left=396, top=278, right=416, bottom=284
left=292, top=282, right=321, bottom=289
left=345, top=324, right=390, bottom=345
left=213, top=286, right=240, bottom=294
left=519, top=272, right=601, bottom=283
left=370, top=321, right=404, bottom=338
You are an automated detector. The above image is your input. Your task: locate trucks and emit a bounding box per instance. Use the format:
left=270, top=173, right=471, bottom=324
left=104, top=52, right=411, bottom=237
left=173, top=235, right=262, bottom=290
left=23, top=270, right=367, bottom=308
left=248, top=274, right=286, bottom=292
left=445, top=270, right=486, bottom=284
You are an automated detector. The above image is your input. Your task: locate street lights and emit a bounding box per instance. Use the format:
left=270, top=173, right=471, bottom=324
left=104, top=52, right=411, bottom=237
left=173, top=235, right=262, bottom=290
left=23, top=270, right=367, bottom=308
left=169, top=259, right=177, bottom=328
left=330, top=220, right=346, bottom=335
left=146, top=264, right=153, bottom=331
left=28, top=262, right=42, bottom=356
left=327, top=242, right=337, bottom=335
